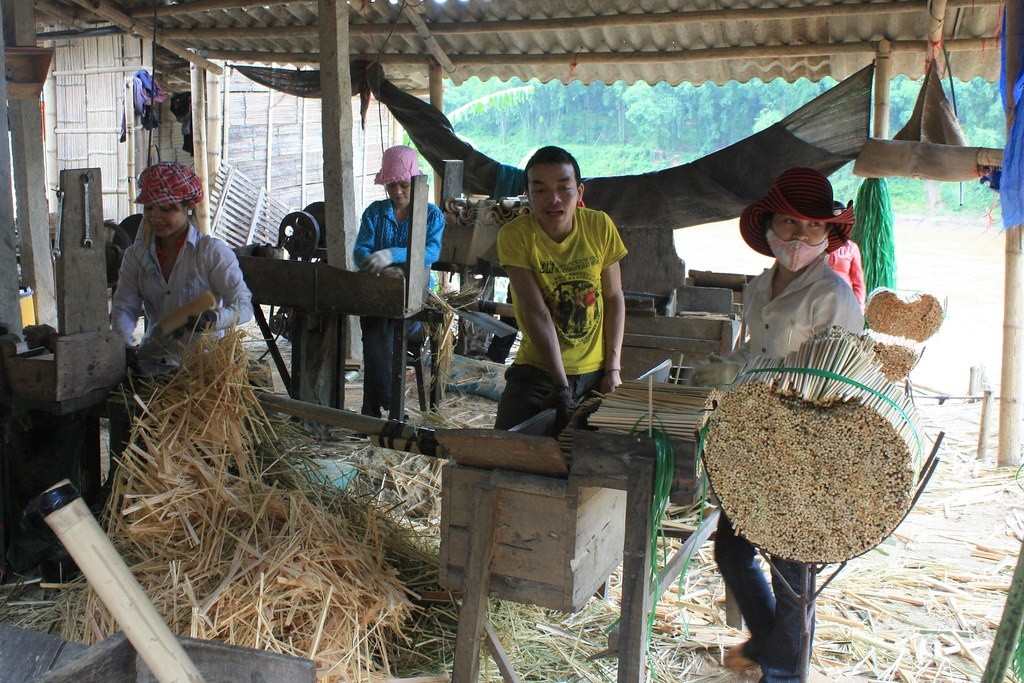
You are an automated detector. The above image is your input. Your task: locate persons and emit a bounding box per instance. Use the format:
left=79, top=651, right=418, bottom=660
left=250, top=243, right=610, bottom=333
left=495, top=146, right=629, bottom=441
left=825, top=201, right=866, bottom=315
left=352, top=145, right=446, bottom=424
left=111, top=161, right=253, bottom=367
left=688, top=166, right=866, bottom=683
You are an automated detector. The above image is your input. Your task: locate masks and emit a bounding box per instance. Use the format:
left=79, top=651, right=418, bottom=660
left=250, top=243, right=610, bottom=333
left=767, top=224, right=829, bottom=272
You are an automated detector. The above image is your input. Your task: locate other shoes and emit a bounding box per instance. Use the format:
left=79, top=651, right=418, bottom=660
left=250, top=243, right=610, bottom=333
left=724, top=643, right=759, bottom=671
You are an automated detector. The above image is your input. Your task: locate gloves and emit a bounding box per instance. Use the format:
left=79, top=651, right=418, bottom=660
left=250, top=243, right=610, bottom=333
left=553, top=383, right=571, bottom=426
left=187, top=311, right=217, bottom=332
left=693, top=352, right=741, bottom=390
left=360, top=248, right=392, bottom=275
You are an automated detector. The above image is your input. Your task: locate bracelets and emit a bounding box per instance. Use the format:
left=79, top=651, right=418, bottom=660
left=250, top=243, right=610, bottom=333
left=602, top=368, right=622, bottom=377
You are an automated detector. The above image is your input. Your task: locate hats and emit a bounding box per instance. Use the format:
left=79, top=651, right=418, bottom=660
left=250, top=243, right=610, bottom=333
left=373, top=145, right=422, bottom=184
left=134, top=161, right=204, bottom=207
left=739, top=166, right=855, bottom=257
